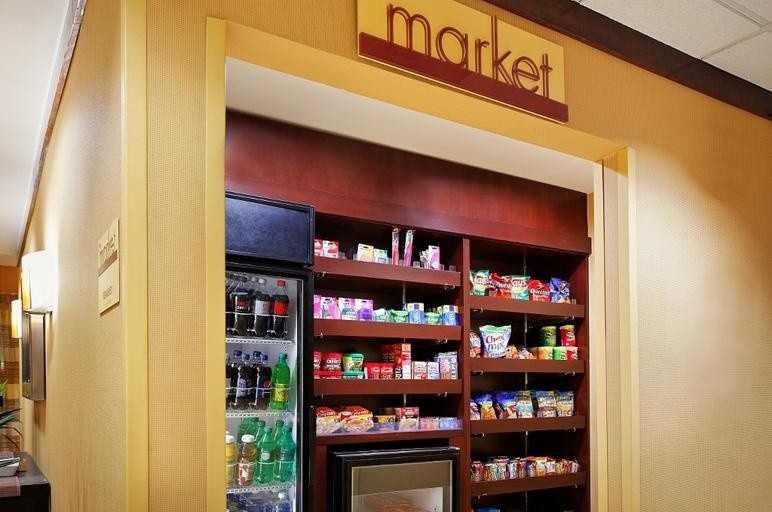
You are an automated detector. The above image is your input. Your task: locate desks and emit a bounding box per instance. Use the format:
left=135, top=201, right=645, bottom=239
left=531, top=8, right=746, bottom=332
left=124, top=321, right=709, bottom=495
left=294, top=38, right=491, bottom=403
left=0, top=451, right=50, bottom=512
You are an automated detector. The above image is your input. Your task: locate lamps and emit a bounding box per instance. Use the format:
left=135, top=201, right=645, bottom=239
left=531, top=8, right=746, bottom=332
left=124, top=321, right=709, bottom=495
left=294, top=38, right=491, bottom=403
left=20, top=249, right=60, bottom=315
left=10, top=298, right=23, bottom=340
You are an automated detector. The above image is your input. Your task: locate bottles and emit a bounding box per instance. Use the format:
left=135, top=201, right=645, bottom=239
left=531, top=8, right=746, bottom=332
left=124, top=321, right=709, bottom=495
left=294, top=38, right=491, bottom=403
left=226, top=352, right=232, bottom=408
left=232, top=353, right=251, bottom=409
left=230, top=349, right=242, bottom=358
left=237, top=421, right=244, bottom=448
left=273, top=420, right=285, bottom=441
left=253, top=421, right=265, bottom=440
left=236, top=494, right=247, bottom=509
left=272, top=280, right=289, bottom=336
left=230, top=494, right=238, bottom=502
left=245, top=490, right=262, bottom=512
left=245, top=418, right=259, bottom=435
left=232, top=276, right=248, bottom=334
left=225, top=435, right=238, bottom=487
left=263, top=491, right=275, bottom=512
left=251, top=279, right=270, bottom=336
left=235, top=435, right=256, bottom=485
left=275, top=426, right=295, bottom=482
left=255, top=427, right=275, bottom=483
left=273, top=492, right=292, bottom=512
left=255, top=355, right=271, bottom=410
left=229, top=277, right=238, bottom=288
left=251, top=276, right=258, bottom=288
left=250, top=351, right=261, bottom=364
left=272, top=352, right=289, bottom=409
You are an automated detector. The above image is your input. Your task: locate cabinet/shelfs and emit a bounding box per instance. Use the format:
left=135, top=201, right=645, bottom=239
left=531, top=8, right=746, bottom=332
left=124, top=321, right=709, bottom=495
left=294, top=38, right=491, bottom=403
left=313, top=208, right=590, bottom=512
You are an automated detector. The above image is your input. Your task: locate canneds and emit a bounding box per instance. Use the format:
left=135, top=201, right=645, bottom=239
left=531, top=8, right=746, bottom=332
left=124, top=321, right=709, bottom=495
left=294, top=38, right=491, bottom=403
left=483, top=464, right=492, bottom=481
left=471, top=460, right=483, bottom=481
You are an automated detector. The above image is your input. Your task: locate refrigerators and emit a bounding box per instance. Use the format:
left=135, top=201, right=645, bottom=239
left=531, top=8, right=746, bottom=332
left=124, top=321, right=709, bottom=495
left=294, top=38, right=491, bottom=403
left=225, top=191, right=315, bottom=512
